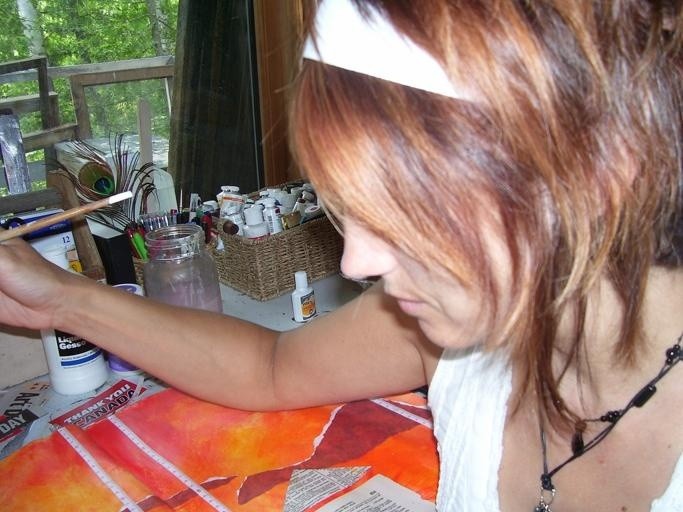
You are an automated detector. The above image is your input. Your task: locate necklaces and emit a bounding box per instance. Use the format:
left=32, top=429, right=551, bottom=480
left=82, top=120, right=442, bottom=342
left=532, top=315, right=682, bottom=509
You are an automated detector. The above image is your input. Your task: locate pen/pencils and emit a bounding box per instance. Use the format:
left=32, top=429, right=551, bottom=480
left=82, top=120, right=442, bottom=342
left=125, top=187, right=213, bottom=261
left=0, top=191, right=133, bottom=241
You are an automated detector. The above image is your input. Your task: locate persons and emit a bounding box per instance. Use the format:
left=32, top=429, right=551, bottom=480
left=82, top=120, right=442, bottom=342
left=0, top=0, right=682, bottom=511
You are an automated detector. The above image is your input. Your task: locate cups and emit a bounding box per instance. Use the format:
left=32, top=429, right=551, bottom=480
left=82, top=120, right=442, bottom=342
left=142, top=224, right=222, bottom=315
